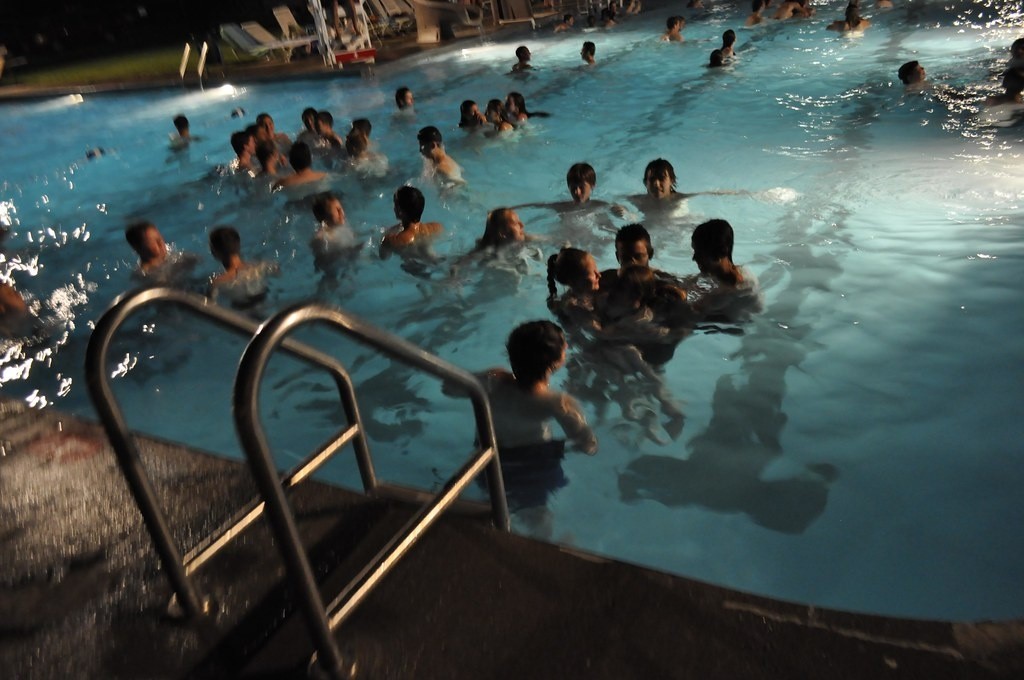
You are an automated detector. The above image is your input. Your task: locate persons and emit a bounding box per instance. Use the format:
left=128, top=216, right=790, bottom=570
left=0, top=0, right=1024, bottom=544
